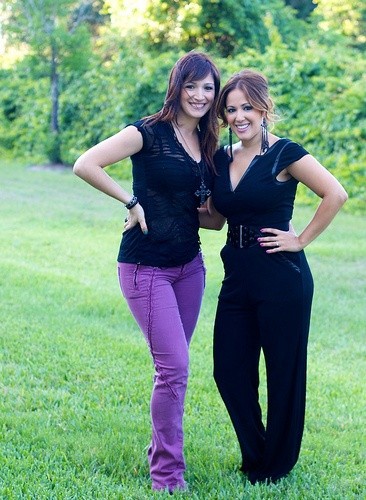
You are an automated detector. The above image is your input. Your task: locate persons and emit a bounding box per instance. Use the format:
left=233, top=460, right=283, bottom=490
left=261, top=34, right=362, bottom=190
left=73, top=51, right=221, bottom=495
left=197, top=71, right=348, bottom=484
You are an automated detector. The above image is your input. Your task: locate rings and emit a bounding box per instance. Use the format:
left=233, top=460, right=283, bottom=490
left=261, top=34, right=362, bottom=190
left=277, top=242, right=278, bottom=246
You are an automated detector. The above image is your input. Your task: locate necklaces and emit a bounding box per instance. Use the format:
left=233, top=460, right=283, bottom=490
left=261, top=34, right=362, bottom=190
left=174, top=123, right=211, bottom=207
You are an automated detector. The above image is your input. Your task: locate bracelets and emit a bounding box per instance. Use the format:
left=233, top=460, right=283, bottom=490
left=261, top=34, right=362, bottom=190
left=128, top=201, right=138, bottom=209
left=124, top=196, right=137, bottom=208
